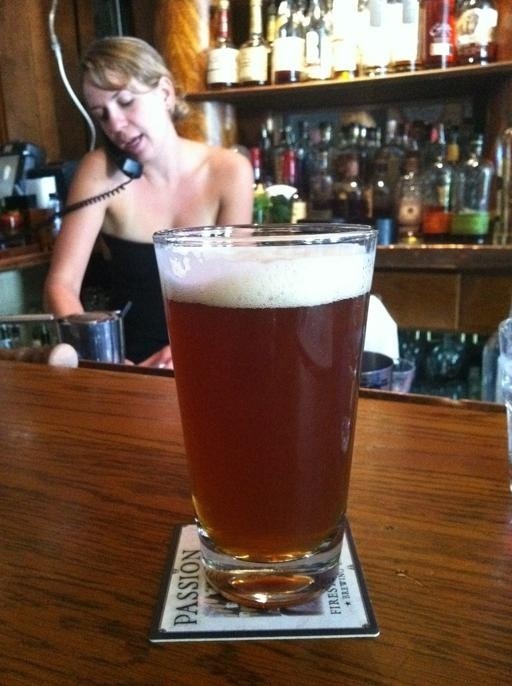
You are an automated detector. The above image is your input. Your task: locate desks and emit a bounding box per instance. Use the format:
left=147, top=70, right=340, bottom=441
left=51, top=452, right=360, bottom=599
left=0, top=354, right=511, bottom=685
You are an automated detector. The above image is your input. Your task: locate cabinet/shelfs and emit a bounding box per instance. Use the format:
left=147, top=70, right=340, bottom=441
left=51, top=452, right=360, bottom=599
left=183, top=1, right=512, bottom=330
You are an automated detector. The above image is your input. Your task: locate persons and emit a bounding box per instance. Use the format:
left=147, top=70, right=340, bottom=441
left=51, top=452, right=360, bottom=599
left=44, top=36, right=253, bottom=371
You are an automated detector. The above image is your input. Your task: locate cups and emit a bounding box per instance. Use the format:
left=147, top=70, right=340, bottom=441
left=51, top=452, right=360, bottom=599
left=359, top=347, right=390, bottom=391
left=53, top=311, right=124, bottom=367
left=152, top=220, right=382, bottom=609
left=391, top=358, right=416, bottom=393
left=497, top=314, right=512, bottom=466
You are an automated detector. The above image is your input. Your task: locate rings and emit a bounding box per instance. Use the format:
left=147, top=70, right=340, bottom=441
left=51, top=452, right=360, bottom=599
left=150, top=361, right=166, bottom=369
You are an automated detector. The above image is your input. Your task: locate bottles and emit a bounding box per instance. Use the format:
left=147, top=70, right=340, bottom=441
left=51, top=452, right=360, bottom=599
left=204, top=0, right=495, bottom=93
left=1, top=323, right=51, bottom=350
left=248, top=111, right=512, bottom=246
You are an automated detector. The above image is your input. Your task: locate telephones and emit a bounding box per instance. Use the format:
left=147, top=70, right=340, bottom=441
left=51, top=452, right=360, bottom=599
left=102, top=130, right=143, bottom=179
